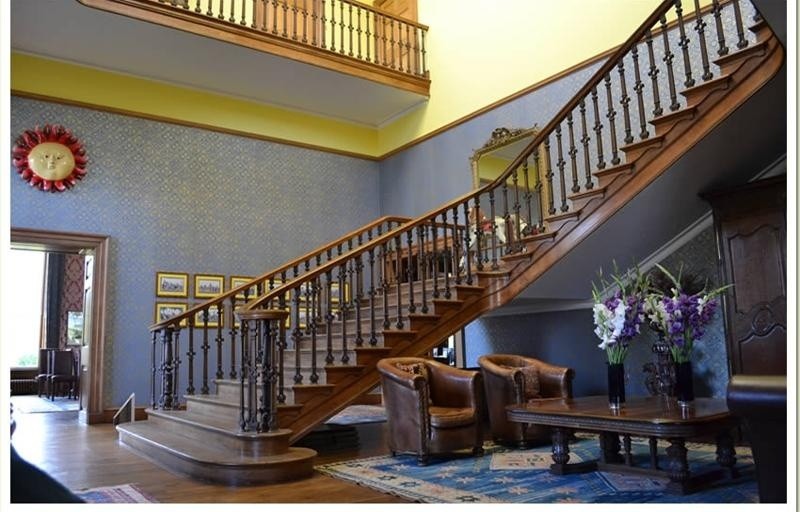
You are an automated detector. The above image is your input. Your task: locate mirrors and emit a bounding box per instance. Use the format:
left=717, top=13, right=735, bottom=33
left=467, top=126, right=554, bottom=236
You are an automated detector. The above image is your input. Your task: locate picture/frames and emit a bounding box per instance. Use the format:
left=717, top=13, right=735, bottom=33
left=154, top=272, right=350, bottom=330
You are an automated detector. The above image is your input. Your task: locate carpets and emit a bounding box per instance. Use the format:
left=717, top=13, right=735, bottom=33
left=315, top=432, right=758, bottom=503
left=71, top=481, right=161, bottom=503
left=10, top=393, right=79, bottom=413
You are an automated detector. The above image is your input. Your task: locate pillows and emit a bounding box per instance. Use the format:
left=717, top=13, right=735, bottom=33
left=396, top=359, right=434, bottom=407
left=499, top=362, right=543, bottom=400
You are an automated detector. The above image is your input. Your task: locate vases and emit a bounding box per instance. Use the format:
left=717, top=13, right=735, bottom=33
left=608, top=360, right=627, bottom=410
left=676, top=361, right=695, bottom=407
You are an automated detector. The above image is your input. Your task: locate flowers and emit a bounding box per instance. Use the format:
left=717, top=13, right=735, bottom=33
left=591, top=258, right=739, bottom=363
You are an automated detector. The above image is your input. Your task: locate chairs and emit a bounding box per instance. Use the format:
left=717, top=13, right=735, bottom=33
left=478, top=353, right=575, bottom=451
left=36, top=347, right=81, bottom=401
left=375, top=355, right=484, bottom=467
